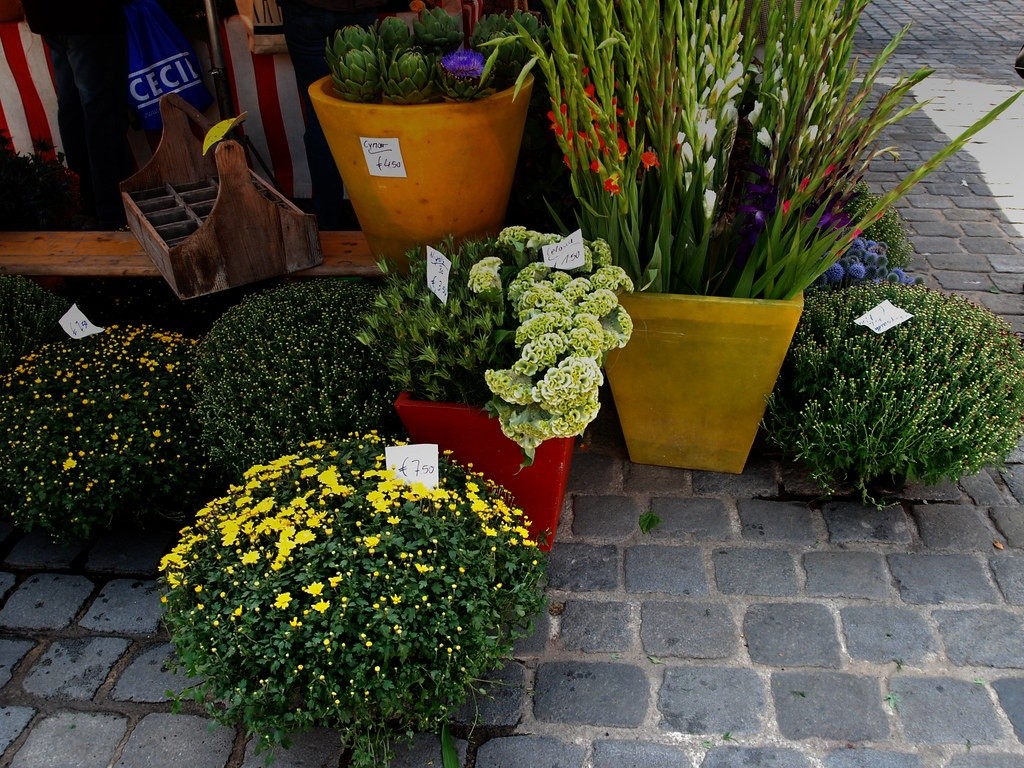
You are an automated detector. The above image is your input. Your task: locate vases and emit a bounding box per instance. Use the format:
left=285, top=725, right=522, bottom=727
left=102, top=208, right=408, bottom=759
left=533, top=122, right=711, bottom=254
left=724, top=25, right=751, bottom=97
left=601, top=291, right=808, bottom=474
left=308, top=76, right=535, bottom=245
left=395, top=394, right=576, bottom=553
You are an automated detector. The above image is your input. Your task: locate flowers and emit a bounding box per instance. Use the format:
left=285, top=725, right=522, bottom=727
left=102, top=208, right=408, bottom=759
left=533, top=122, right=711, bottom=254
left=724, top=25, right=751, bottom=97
left=153, top=432, right=552, bottom=768
left=324, top=10, right=507, bottom=103
left=354, top=223, right=640, bottom=469
left=478, top=0, right=1023, bottom=296
left=758, top=277, right=1024, bottom=512
left=0, top=324, right=200, bottom=544
left=184, top=278, right=400, bottom=483
